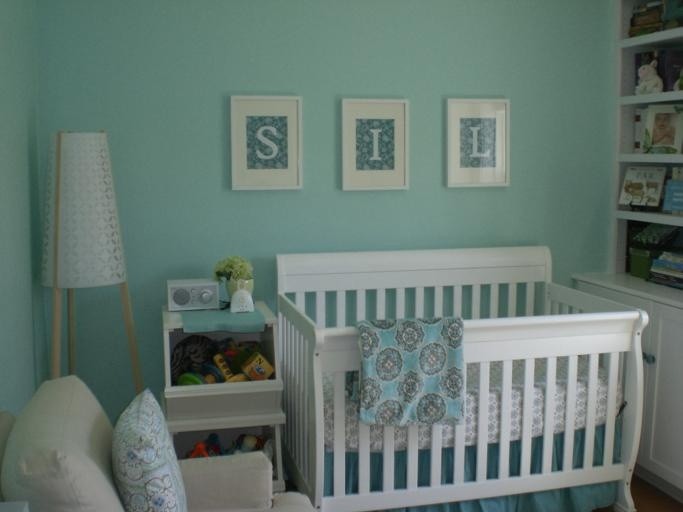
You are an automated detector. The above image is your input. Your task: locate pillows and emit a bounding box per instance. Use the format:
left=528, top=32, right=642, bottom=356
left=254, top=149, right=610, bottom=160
left=112, top=386, right=187, bottom=512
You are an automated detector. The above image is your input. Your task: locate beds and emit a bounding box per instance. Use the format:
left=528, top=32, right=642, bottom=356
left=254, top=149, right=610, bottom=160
left=272, top=245, right=651, bottom=511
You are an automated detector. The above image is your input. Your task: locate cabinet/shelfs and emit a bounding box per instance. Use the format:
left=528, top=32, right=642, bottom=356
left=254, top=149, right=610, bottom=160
left=570, top=272, right=682, bottom=502
left=610, top=1, right=682, bottom=288
left=155, top=297, right=285, bottom=492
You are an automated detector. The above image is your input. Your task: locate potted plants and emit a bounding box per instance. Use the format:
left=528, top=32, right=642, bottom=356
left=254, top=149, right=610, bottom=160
left=214, top=253, right=253, bottom=304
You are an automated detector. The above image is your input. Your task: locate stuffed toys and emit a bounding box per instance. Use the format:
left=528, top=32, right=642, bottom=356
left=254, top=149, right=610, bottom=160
left=634, top=60, right=662, bottom=93
left=173, top=339, right=274, bottom=385
left=181, top=431, right=265, bottom=459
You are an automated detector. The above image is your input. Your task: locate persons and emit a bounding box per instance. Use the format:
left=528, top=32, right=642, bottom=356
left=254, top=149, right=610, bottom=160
left=652, top=114, right=675, bottom=145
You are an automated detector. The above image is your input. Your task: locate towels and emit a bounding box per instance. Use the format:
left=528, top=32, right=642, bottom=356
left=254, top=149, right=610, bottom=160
left=354, top=312, right=465, bottom=427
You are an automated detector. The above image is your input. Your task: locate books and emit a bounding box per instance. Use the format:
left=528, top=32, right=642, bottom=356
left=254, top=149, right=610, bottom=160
left=644, top=252, right=683, bottom=290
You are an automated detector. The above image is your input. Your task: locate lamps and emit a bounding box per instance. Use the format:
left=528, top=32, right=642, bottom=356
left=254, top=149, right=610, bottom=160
left=36, top=130, right=150, bottom=389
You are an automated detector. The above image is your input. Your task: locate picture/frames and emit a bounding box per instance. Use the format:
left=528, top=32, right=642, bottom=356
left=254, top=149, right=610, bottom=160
left=228, top=92, right=302, bottom=196
left=445, top=98, right=510, bottom=189
left=338, top=97, right=409, bottom=194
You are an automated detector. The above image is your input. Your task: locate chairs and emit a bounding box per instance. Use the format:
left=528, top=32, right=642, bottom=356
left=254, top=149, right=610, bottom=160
left=0, top=376, right=314, bottom=512
